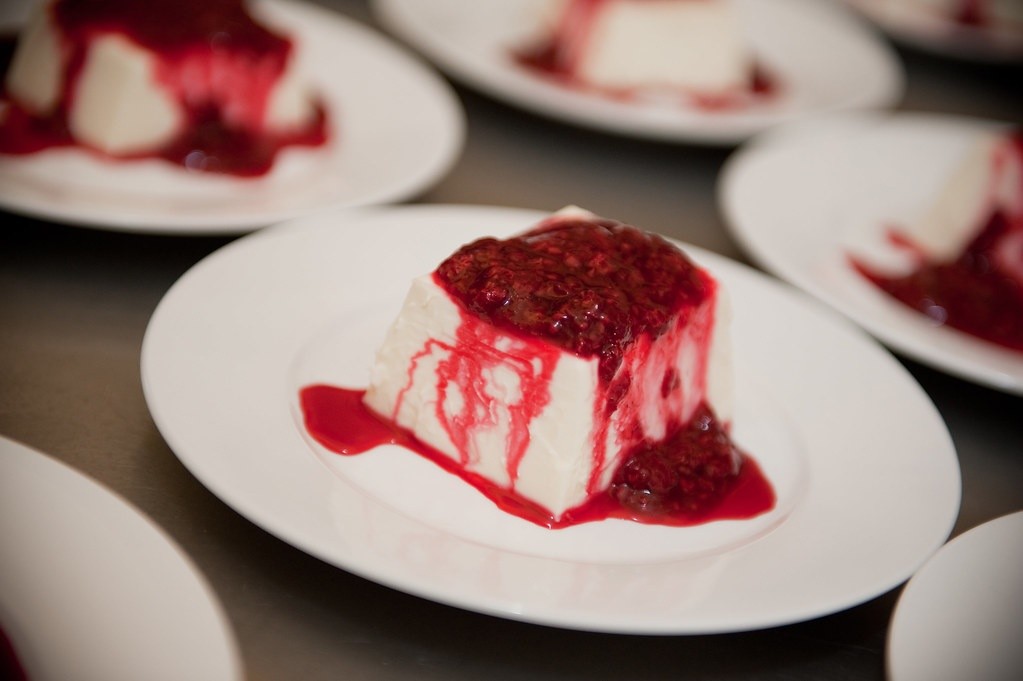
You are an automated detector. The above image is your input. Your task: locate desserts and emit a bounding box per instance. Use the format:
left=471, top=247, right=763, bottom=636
left=358, top=200, right=722, bottom=528
left=552, top=0, right=764, bottom=103
left=915, top=117, right=1023, bottom=342
left=9, top=1, right=326, bottom=161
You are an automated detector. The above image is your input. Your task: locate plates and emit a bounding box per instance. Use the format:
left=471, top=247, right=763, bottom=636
left=139, top=202, right=963, bottom=635
left=378, top=1, right=903, bottom=146
left=0, top=436, right=244, bottom=681
left=0, top=1, right=468, bottom=236
left=886, top=512, right=1023, bottom=681
left=860, top=0, right=1023, bottom=57
left=716, top=110, right=1023, bottom=396
